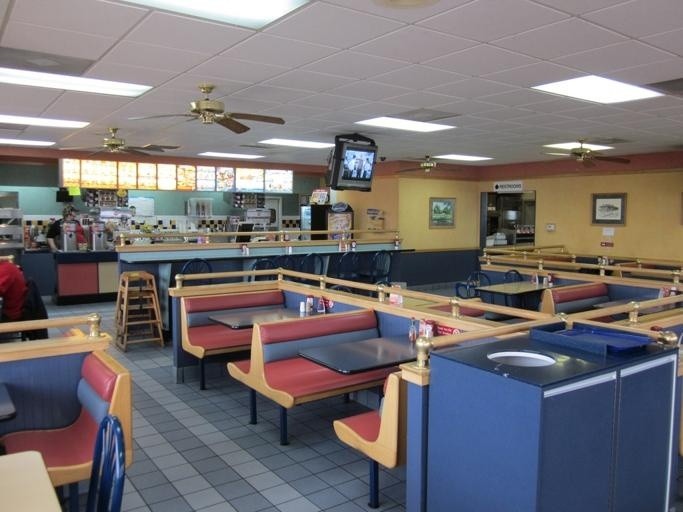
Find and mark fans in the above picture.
[127,82,286,136]
[538,138,630,162]
[395,155,462,175]
[58,127,180,157]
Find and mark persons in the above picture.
[341,154,372,181]
[46,205,87,254]
[130,206,137,218]
[0,259,28,322]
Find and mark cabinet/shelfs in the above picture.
[0,206,24,250]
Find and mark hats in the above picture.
[63,206,80,216]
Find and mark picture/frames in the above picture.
[429,197,457,228]
[592,193,627,224]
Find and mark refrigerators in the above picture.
[299,204,354,240]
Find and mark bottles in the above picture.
[280,231,289,241]
[670,286,677,297]
[543,272,553,287]
[409,317,434,342]
[242,244,249,255]
[394,235,399,249]
[299,294,326,313]
[390,285,402,304]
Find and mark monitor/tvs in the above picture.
[327,141,378,188]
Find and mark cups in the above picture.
[351,241,357,250]
